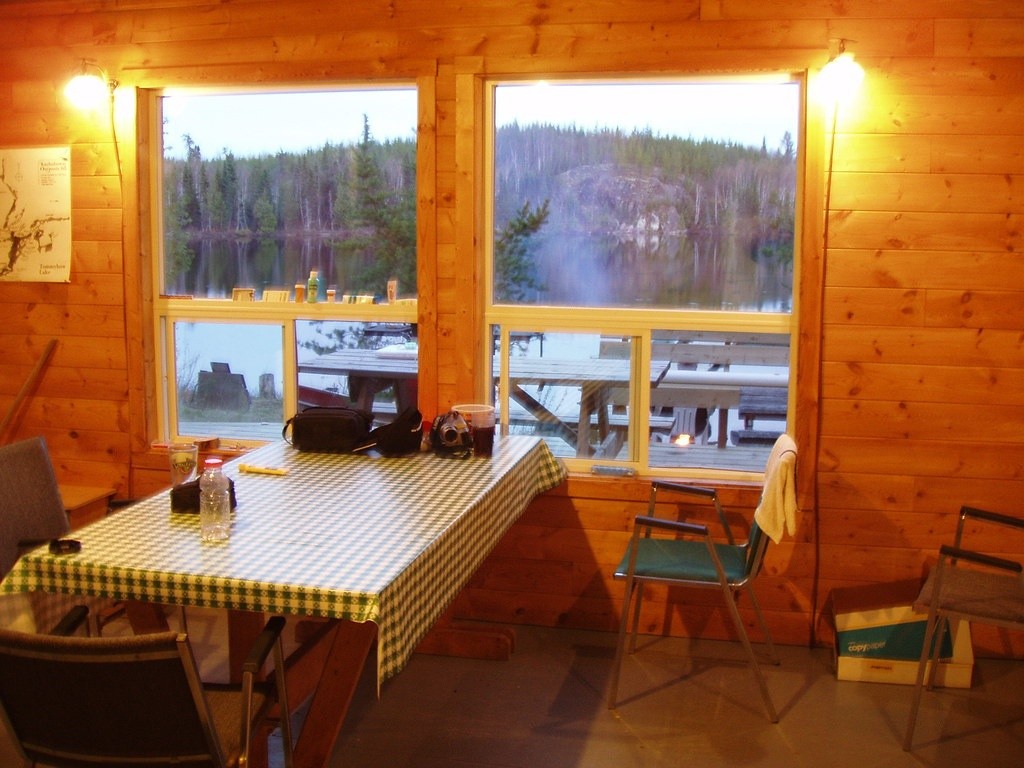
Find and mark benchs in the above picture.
[586,325,791,461]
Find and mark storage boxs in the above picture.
[832,576,955,660]
[833,616,974,690]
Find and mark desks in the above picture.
[297,348,673,460]
[0,434,570,768]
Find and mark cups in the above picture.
[168,445,198,491]
[451,404,495,447]
[294,285,306,303]
[472,412,495,458]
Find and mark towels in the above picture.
[753,433,799,544]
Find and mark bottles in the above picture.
[307,271,320,304]
[387,280,397,305]
[326,290,336,303]
[200,458,231,546]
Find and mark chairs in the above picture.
[0,435,295,768]
[902,506,1023,753]
[606,433,799,723]
